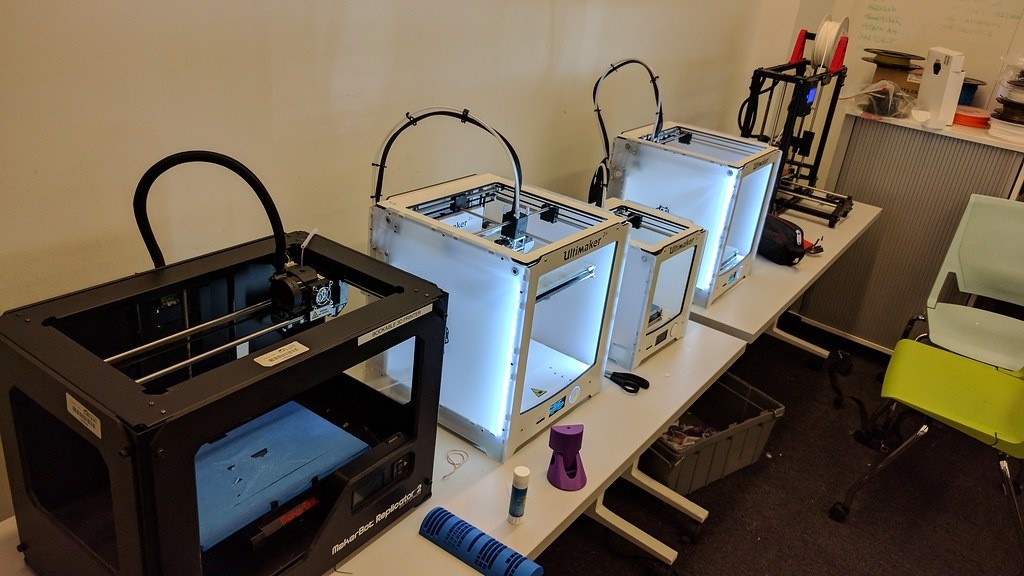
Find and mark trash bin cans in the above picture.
[645,370,786,496]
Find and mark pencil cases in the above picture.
[757,214,805,267]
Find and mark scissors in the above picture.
[603,371,649,394]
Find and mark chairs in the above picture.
[830,339,1024,543]
[880,194,1024,495]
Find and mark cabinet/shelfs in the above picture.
[788,115,1024,356]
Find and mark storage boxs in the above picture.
[637,371,785,495]
[915,47,966,125]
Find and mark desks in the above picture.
[0,320,748,576]
[689,185,883,359]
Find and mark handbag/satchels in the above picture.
[757,212,805,267]
